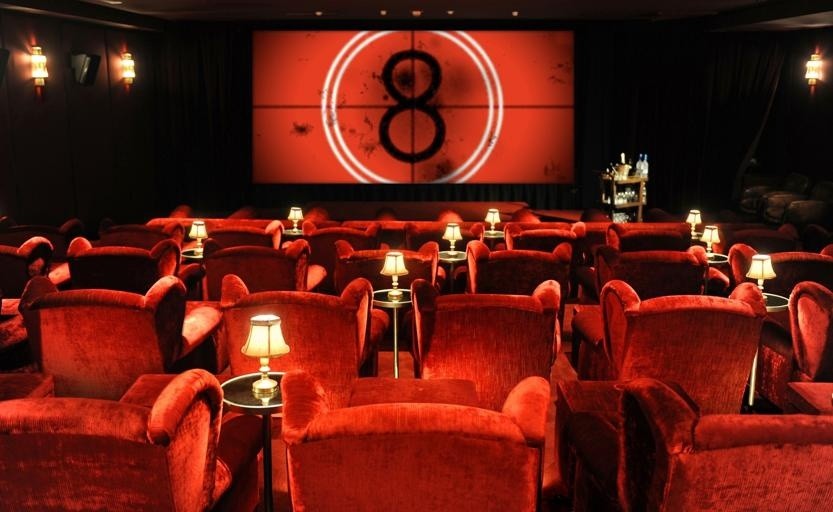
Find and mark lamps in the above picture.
[0,49,10,75]
[804,54,832,88]
[287,206,304,232]
[30,45,49,87]
[120,51,137,84]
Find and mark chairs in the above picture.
[1,210,833,511]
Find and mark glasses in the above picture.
[287,206,304,232]
[120,51,137,84]
[0,49,10,75]
[30,45,49,87]
[804,54,832,88]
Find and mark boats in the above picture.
[70,53,101,87]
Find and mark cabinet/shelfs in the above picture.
[607,152,651,180]
[602,173,649,222]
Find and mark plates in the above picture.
[602,173,649,222]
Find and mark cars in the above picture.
[608,185,646,225]
